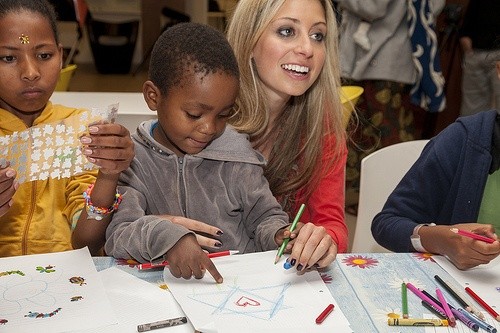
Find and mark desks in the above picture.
[49,89,160,137]
[2,251,500,331]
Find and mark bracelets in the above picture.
[84,183,124,221]
[411,222,437,254]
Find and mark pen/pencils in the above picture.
[401,274,500,333]
[134,250,242,272]
[450,228,495,243]
[274,204,307,265]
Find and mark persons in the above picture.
[1,0,136,259]
[371,110,500,271]
[154,1,360,276]
[103,22,307,284]
[336,0,447,215]
[458,0,500,120]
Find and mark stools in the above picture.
[85,16,141,76]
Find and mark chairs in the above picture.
[348,136,440,255]
